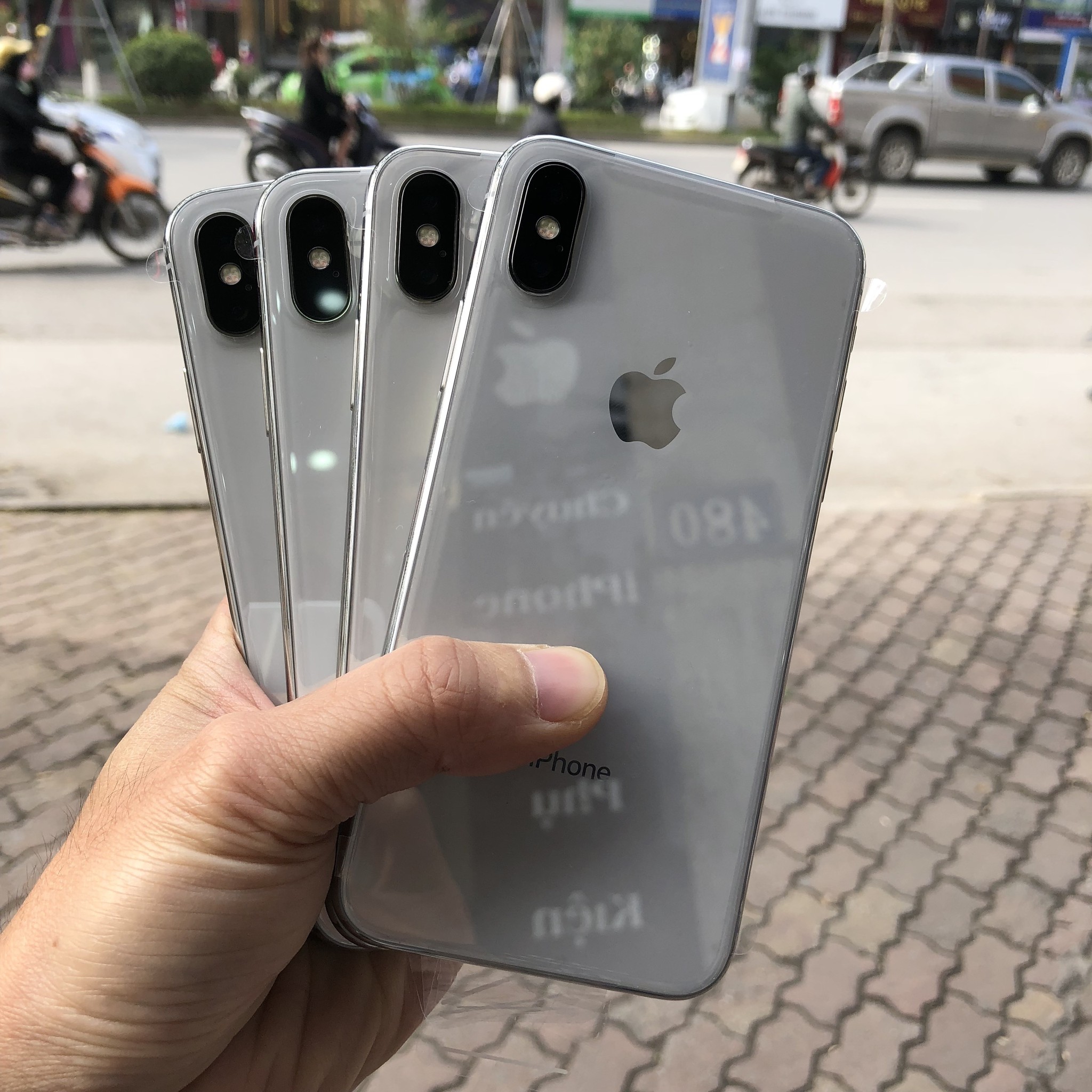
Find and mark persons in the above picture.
[0,37,87,241]
[0,591,608,1092]
[522,73,571,139]
[299,38,361,168]
[782,63,843,200]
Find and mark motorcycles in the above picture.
[0,118,174,264]
[239,91,400,184]
[735,121,874,219]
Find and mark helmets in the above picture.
[533,72,570,104]
[0,34,31,69]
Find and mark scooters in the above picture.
[206,56,283,106]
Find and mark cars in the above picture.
[33,91,162,193]
[276,42,460,117]
[776,49,1092,193]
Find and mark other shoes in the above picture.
[32,221,71,242]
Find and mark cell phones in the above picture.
[328,134,867,1001]
[161,134,502,955]
